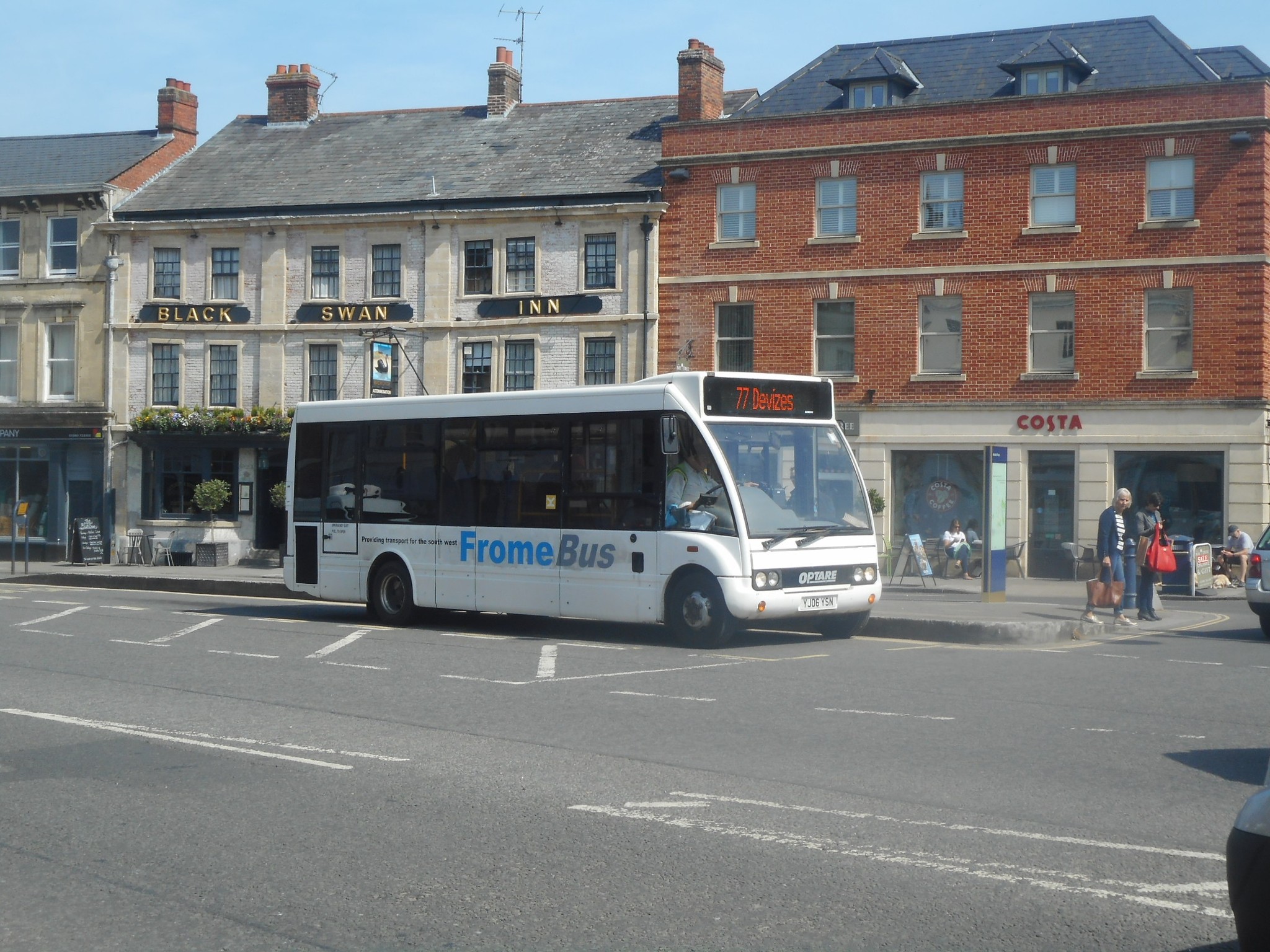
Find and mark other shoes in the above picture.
[955,563,960,569]
[1080,614,1103,624]
[1114,616,1137,627]
[1237,580,1245,587]
[963,575,973,580]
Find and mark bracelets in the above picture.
[1233,553,1235,558]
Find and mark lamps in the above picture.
[1225,128,1255,147]
[668,167,690,179]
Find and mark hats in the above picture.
[1227,525,1238,536]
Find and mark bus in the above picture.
[278,371,883,650]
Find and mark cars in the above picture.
[1244,522,1270,637]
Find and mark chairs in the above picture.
[882,534,897,578]
[1059,541,1096,580]
[1005,540,1027,579]
[150,530,177,568]
[878,553,890,579]
[943,556,971,580]
[909,536,943,579]
[123,528,146,565]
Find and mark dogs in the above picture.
[1195,573,1237,589]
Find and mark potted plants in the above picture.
[190,478,232,567]
[268,481,286,567]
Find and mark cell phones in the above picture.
[1160,520,1165,524]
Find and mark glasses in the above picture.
[953,526,960,528]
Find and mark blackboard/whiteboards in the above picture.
[69,517,105,563]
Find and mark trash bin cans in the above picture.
[1161,535,1194,595]
[165,551,194,567]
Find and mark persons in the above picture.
[784,467,796,511]
[1080,488,1137,627]
[1218,525,1254,587]
[942,518,973,579]
[964,519,979,549]
[1134,491,1167,622]
[664,443,760,518]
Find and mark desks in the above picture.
[125,534,155,566]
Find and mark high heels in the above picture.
[1137,611,1156,621]
[1149,609,1162,621]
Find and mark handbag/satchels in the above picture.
[1085,563,1125,608]
[1146,523,1176,573]
